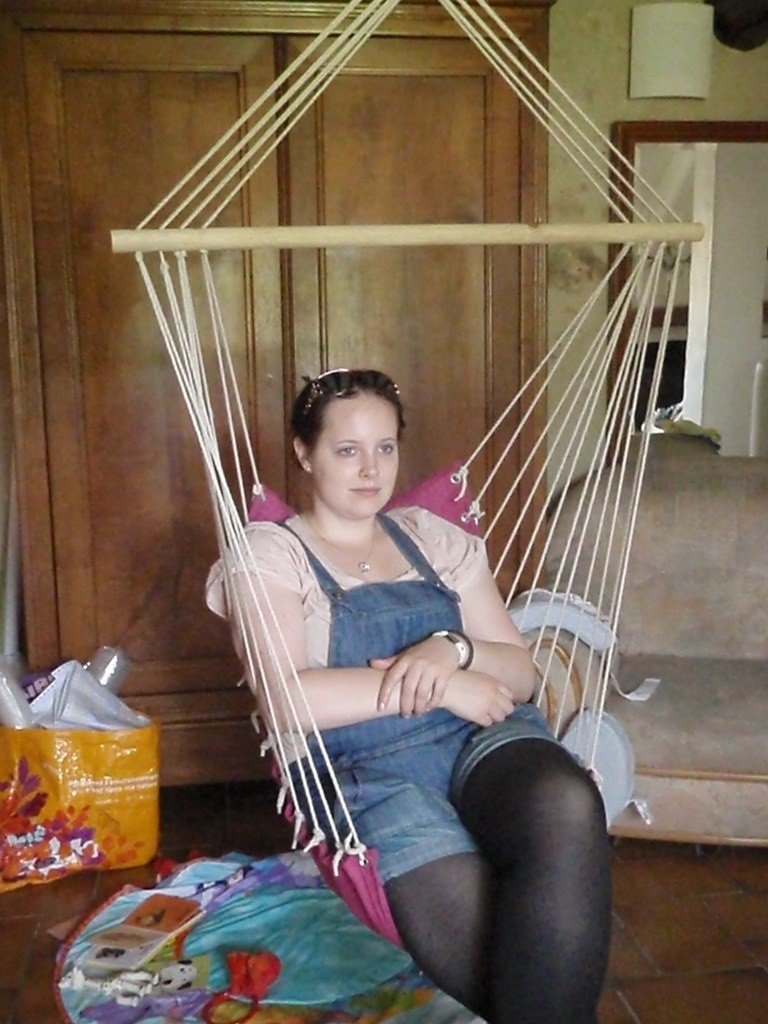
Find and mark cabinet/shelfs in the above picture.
[0,0,555,791]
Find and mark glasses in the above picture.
[296,369,403,433]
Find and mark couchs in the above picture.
[514,455,767,853]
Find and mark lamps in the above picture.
[628,2,716,99]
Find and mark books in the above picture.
[83,894,204,970]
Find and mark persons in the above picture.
[206,370,613,1024]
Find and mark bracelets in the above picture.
[433,630,473,669]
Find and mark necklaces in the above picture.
[307,517,376,572]
